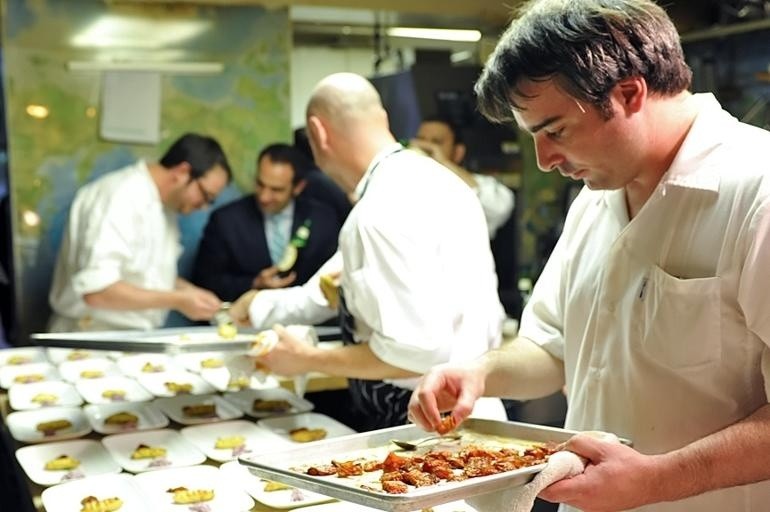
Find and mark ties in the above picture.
[270,214,284,264]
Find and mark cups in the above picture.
[214,302,242,341]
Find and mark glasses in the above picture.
[194,179,215,205]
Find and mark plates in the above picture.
[255,417,358,444]
[2,345,279,411]
[41,465,256,512]
[15,438,123,485]
[179,420,291,464]
[6,388,312,445]
[101,428,206,473]
[218,462,365,512]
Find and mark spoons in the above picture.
[390,433,466,453]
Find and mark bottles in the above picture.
[276,217,314,277]
[517,275,538,309]
[400,137,434,160]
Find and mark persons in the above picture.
[43,130,236,337]
[197,66,515,422]
[407,0,770,512]
